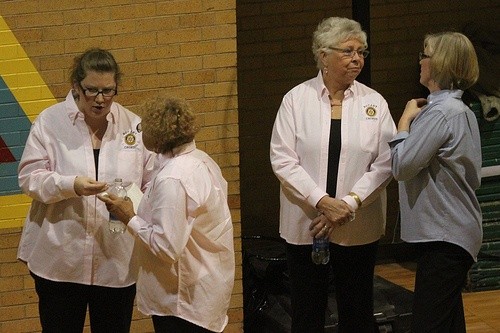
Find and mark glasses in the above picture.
[326,47,369,59]
[418,53,432,59]
[136,123,144,132]
[78,82,118,97]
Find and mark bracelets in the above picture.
[348,192,363,207]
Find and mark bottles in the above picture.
[108,178,127,234]
[311,234,330,264]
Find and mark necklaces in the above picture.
[331,104,342,113]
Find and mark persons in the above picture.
[388,31,483,333]
[16,46,157,333]
[96,92,236,333]
[270,17,398,333]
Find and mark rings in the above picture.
[324,226,327,229]
